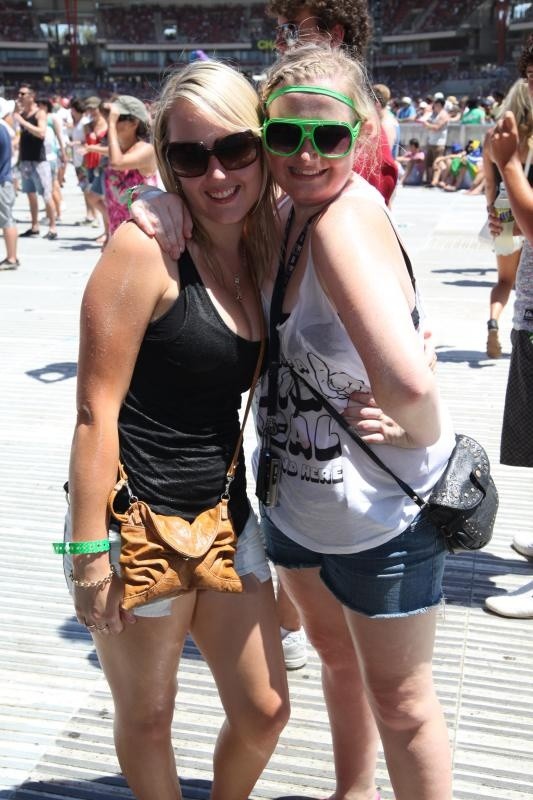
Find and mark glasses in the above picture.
[167,131,259,177]
[262,117,361,158]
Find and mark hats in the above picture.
[1,97,15,119]
[84,96,101,109]
[103,96,148,123]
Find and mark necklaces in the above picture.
[218,260,250,308]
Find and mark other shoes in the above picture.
[487,318,502,359]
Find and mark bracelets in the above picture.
[70,565,117,588]
[126,180,142,213]
[53,541,112,555]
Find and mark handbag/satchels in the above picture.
[120,501,243,610]
[428,435,498,551]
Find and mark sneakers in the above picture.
[485,581,533,618]
[76,218,92,226]
[90,218,100,229]
[281,626,307,669]
[512,534,533,557]
[41,231,58,242]
[20,229,39,237]
[0,259,20,271]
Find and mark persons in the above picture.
[482,53,533,622]
[252,0,398,671]
[123,42,454,800]
[0,85,167,271]
[352,84,500,196]
[62,60,290,800]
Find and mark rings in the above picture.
[86,625,96,633]
[98,627,107,632]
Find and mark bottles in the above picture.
[492,182,514,256]
